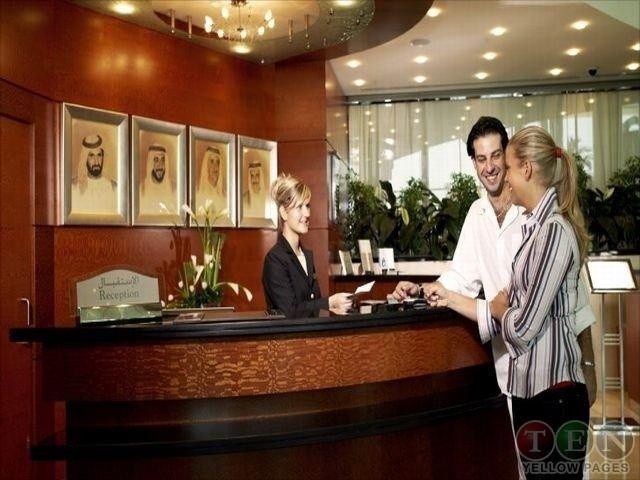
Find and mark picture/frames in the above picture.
[59,101,280,230]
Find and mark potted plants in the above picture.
[335,138,640,261]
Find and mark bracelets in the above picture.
[418,283,424,298]
[582,360,595,367]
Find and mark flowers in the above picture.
[159,201,253,309]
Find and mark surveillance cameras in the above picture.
[588,67,598,76]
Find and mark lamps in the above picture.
[204,1,276,40]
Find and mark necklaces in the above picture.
[495,197,510,218]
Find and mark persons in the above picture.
[260,173,355,315]
[244,160,268,218]
[195,145,228,216]
[387,115,598,408]
[423,126,588,479]
[139,143,177,215]
[72,134,118,213]
